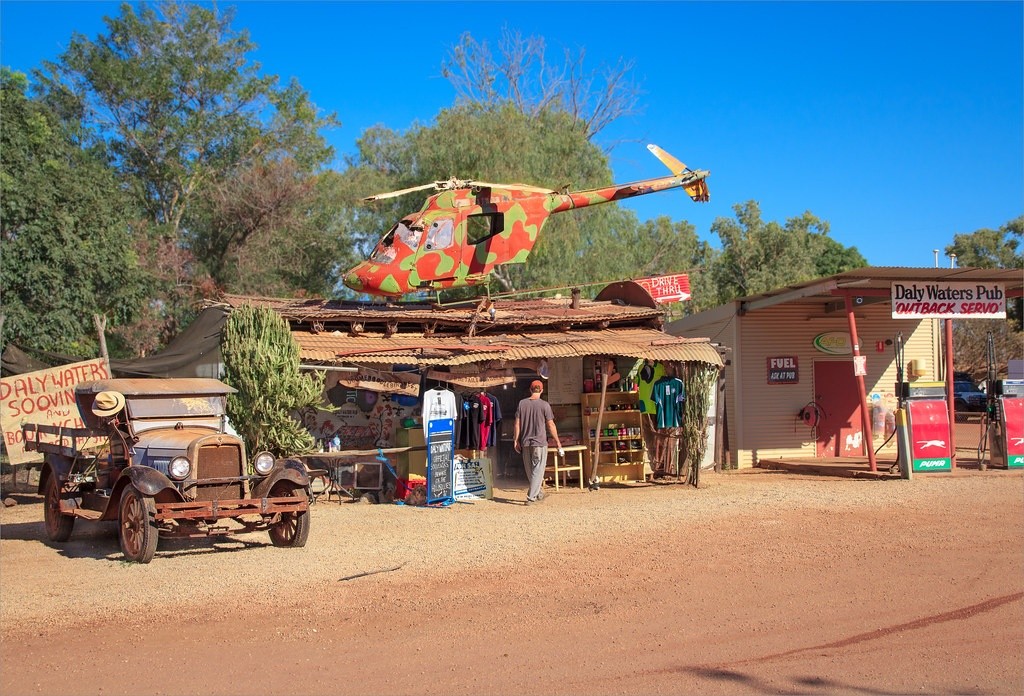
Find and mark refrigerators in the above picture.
[395,428,428,480]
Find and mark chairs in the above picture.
[300,457,328,505]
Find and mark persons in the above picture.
[513,380,565,505]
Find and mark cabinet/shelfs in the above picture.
[581,392,646,484]
[396,428,426,479]
[584,356,615,392]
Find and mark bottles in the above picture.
[316,435,340,453]
[596,369,600,373]
[618,378,638,391]
[596,359,601,366]
[596,374,601,381]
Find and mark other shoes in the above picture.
[538,493,549,501]
[525,499,533,505]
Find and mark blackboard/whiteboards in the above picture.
[428,435,454,502]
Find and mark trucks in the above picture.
[20,378,315,564]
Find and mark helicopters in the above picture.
[342,142,711,323]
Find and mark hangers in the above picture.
[434,381,487,397]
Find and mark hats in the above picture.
[531,380,543,390]
[92,391,125,416]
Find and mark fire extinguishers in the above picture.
[873,406,886,433]
[886,409,897,438]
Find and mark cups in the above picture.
[631,404,637,410]
[610,405,618,410]
[620,404,627,410]
[590,423,640,436]
[627,404,632,410]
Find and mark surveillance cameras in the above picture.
[856,297,863,303]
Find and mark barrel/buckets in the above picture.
[583,378,594,393]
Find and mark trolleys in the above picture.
[374,448,428,508]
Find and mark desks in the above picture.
[545,445,587,491]
[501,438,519,478]
[294,447,411,505]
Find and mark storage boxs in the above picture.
[396,479,427,500]
[355,462,382,490]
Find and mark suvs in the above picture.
[954,380,988,424]
